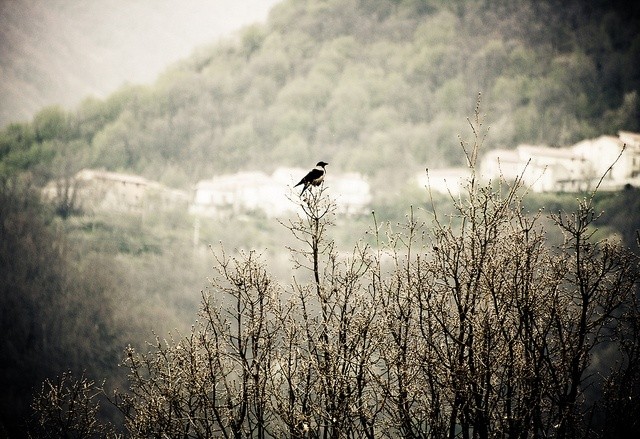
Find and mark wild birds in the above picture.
[294,161,330,197]
